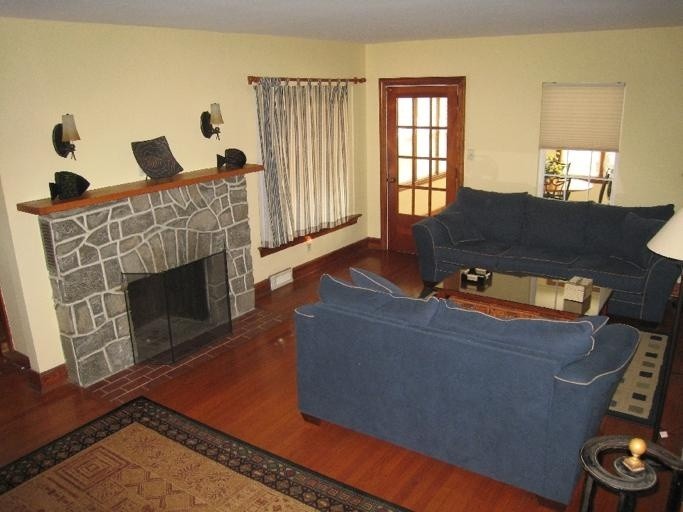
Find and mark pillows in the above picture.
[521,194,588,252]
[589,203,675,258]
[457,185,528,248]
[428,297,595,359]
[609,211,666,272]
[319,273,439,329]
[568,315,609,335]
[349,267,402,297]
[432,212,486,246]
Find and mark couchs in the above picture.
[411,186,683,324]
[293,274,642,505]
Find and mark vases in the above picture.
[544,176,560,192]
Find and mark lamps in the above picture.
[647,207,682,442]
[200,102,224,141]
[52,113,80,160]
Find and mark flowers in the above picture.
[543,148,564,176]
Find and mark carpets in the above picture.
[0,395,421,512]
[607,328,673,427]
